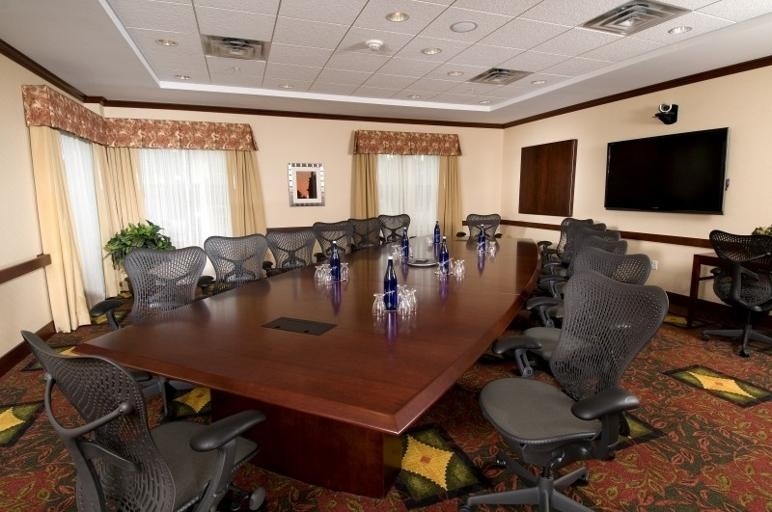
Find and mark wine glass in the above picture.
[476,241,496,259]
[391,244,405,261]
[435,257,465,278]
[313,262,349,283]
[373,284,420,318]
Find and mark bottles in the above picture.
[402,227,411,258]
[433,243,440,261]
[477,250,486,280]
[382,255,399,310]
[401,263,410,283]
[433,221,440,243]
[439,276,451,310]
[385,311,397,343]
[329,240,342,282]
[478,223,485,252]
[331,285,341,316]
[439,235,450,274]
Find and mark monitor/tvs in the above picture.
[604,126,730,216]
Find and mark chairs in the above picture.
[21,328,284,512]
[703,230,772,356]
[108,214,411,381]
[457,214,671,512]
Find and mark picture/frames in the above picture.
[288,162,325,206]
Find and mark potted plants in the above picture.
[102,219,175,298]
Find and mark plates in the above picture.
[406,258,436,267]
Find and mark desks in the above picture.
[686,255,771,327]
[72,236,541,500]
